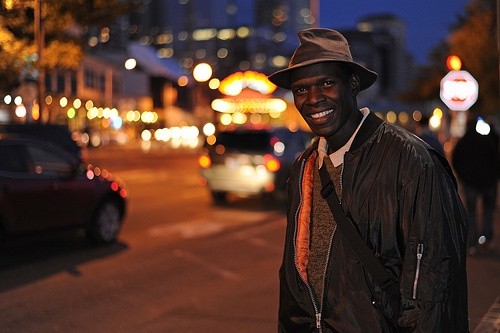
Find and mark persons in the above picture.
[413,116,444,153]
[269,28,469,333]
[452,110,500,256]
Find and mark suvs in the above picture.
[199,128,307,206]
[0,124,126,247]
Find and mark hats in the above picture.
[268,27,378,92]
